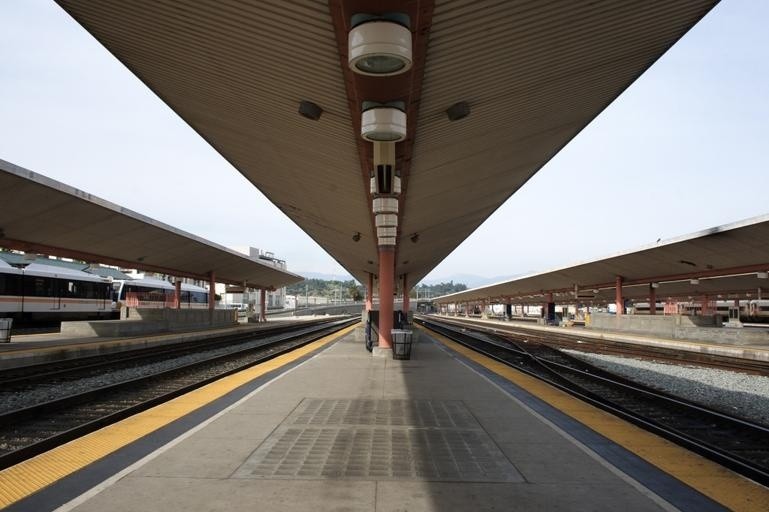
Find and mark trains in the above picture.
[2,257,220,332]
[626,298,769,327]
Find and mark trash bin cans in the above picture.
[390,329,414,360]
[0,318,14,343]
